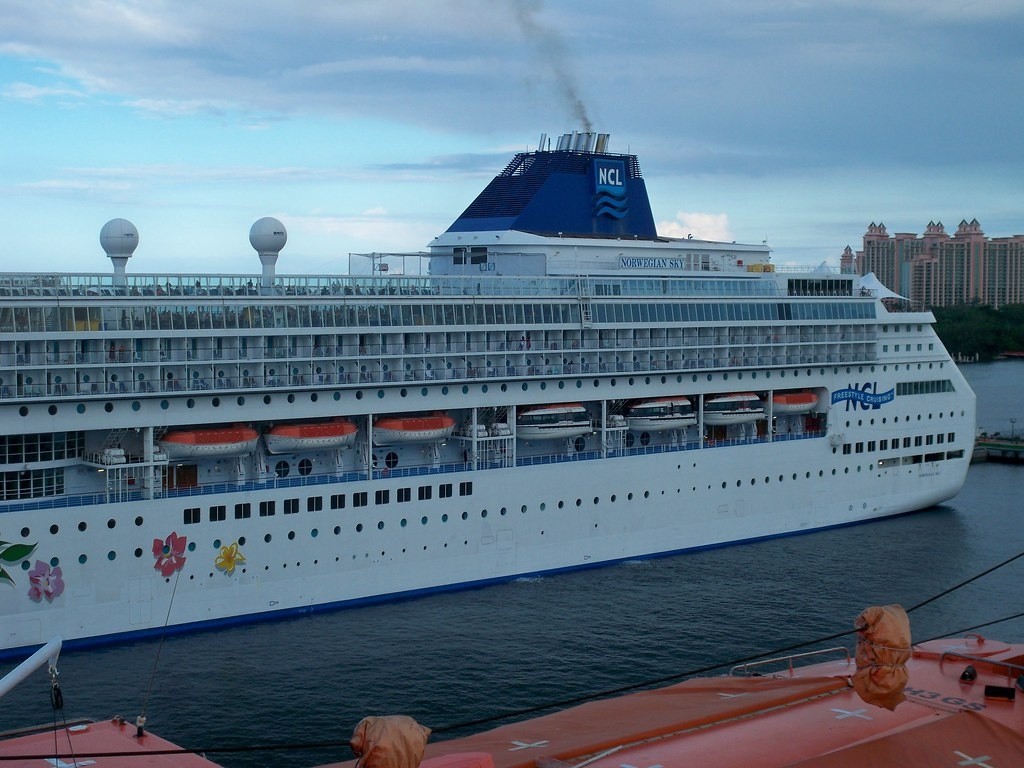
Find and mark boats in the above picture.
[515,402,594,440]
[622,396,698,429]
[371,414,457,444]
[2,129,978,655]
[771,392,820,415]
[703,393,764,423]
[149,421,260,459]
[261,420,360,454]
[970,417,1024,462]
[0,603,1023,768]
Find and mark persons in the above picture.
[518,336,525,351]
[526,335,531,350]
[508,336,514,350]
[76,279,492,330]
[107,345,116,362]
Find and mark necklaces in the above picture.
[117,345,127,363]
